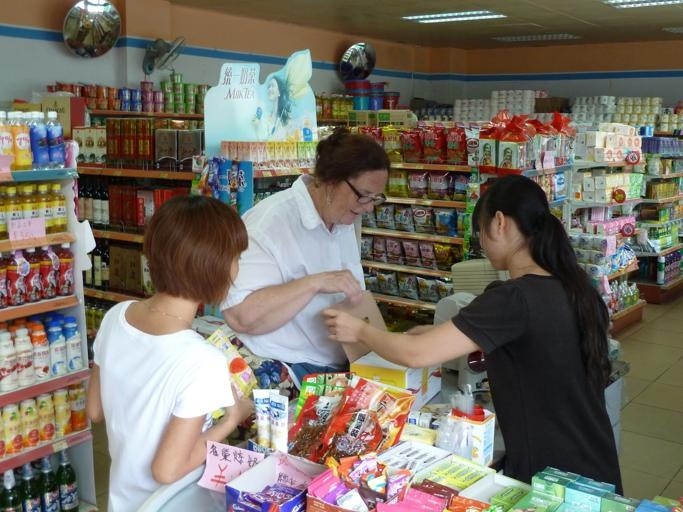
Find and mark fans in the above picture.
[142,35,187,75]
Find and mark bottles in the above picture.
[312,92,354,121]
[609,279,641,315]
[0,111,111,512]
[633,218,683,288]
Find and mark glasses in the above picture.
[343,178,386,206]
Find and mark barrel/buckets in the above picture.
[346,79,400,109]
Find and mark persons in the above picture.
[82,193,256,512]
[319,170,625,501]
[264,52,315,132]
[216,125,391,394]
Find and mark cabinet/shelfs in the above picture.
[57,108,572,335]
[566,112,681,337]
[0,109,95,512]
[136,335,631,512]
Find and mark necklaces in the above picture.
[139,299,190,326]
